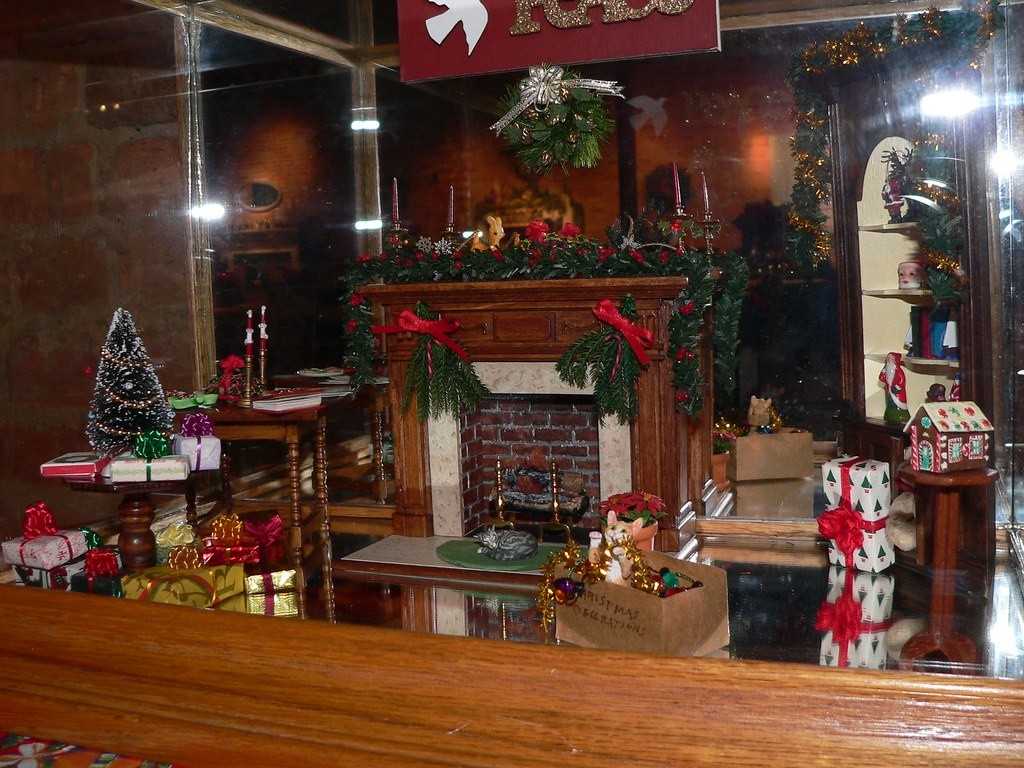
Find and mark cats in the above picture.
[588,510,645,589]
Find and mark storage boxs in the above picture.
[120,563,244,608]
[102,451,190,484]
[167,394,218,410]
[553,551,730,656]
[821,457,895,575]
[11,545,123,591]
[40,452,111,477]
[726,428,815,481]
[71,568,126,597]
[244,569,297,595]
[245,592,299,618]
[1,529,88,570]
[172,434,221,471]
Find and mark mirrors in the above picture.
[187,0,1014,562]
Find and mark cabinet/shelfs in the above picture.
[828,64,993,466]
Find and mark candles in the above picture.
[700,171,709,210]
[671,159,681,206]
[446,186,454,224]
[257,305,268,349]
[244,310,254,355]
[393,177,399,221]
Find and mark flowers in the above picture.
[594,488,668,529]
[712,428,737,455]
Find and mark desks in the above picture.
[60,472,202,570]
[172,400,336,621]
[273,374,391,504]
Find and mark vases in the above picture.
[711,452,730,493]
[599,520,658,552]
